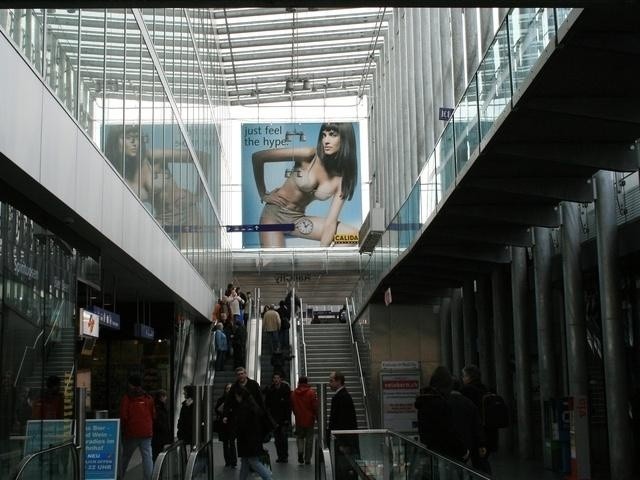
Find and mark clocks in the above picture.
[299,220,314,233]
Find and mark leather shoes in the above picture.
[282,458,288,463]
[276,457,282,462]
[298,455,303,463]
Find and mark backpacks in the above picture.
[479,391,509,428]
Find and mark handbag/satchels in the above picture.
[249,445,271,472]
[213,413,223,433]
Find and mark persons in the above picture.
[413,361,492,480]
[328,370,361,480]
[105,124,211,249]
[208,282,302,480]
[250,124,360,248]
[0,368,71,478]
[86,314,96,334]
[120,382,198,480]
[291,376,318,465]
